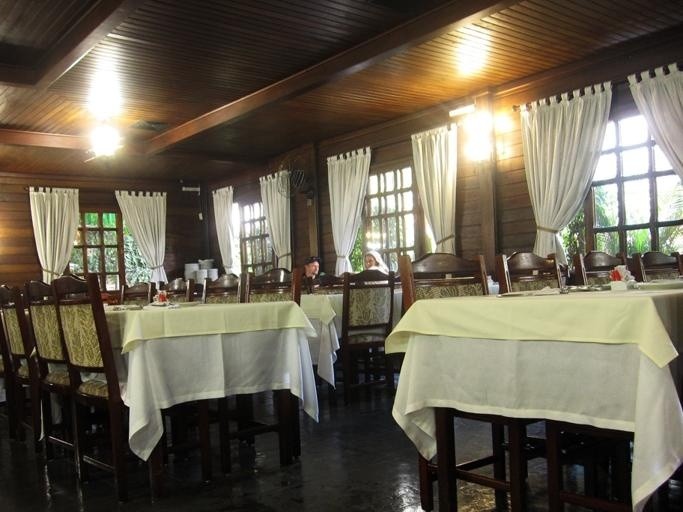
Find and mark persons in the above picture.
[300,256,321,289]
[364,250,389,273]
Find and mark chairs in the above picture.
[197,266,305,474]
[633,252,682,283]
[0,284,44,453]
[52,273,211,503]
[399,254,563,512]
[498,251,628,481]
[573,251,630,286]
[22,279,103,473]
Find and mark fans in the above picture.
[276,154,314,200]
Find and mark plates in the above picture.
[184,263,219,284]
